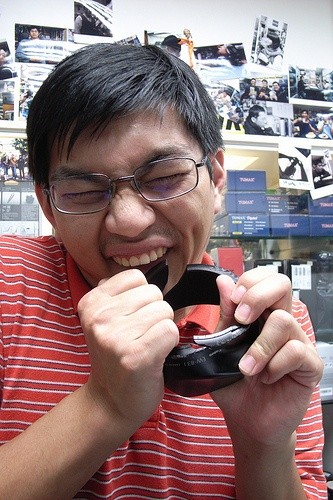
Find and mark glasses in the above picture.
[42,155,208,215]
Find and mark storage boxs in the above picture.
[214,169,333,235]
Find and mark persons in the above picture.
[0,40,329,500]
[163,26,333,190]
[75,5,99,37]
[20,26,46,62]
[0,41,18,80]
[4,156,24,181]
